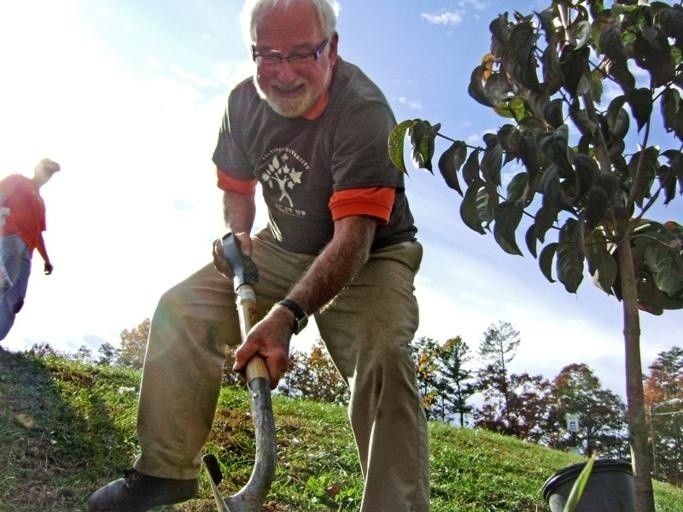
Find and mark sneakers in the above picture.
[86,467,200,512]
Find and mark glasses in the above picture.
[250,37,331,70]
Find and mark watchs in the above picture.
[278,298,308,335]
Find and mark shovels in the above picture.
[200,232,276,512]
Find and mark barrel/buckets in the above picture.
[541,457,638,511]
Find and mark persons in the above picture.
[0,159,61,341]
[87,1,431,511]
